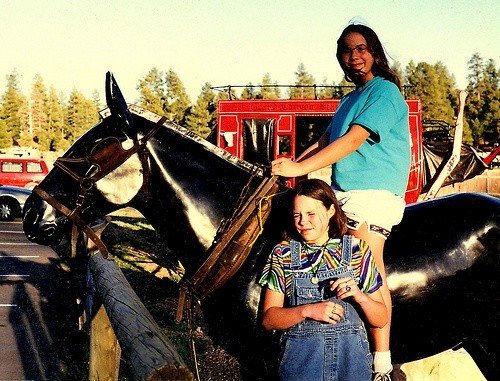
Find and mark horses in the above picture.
[23,71,500,381]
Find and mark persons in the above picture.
[271,24,411,381]
[259,178,388,381]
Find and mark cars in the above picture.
[0,184,33,221]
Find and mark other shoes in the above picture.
[373,365,393,381]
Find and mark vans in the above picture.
[0,157,49,191]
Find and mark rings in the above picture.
[330,313,334,318]
[334,302,336,306]
[345,282,348,287]
[346,287,351,292]
[327,318,331,323]
[332,307,336,313]
